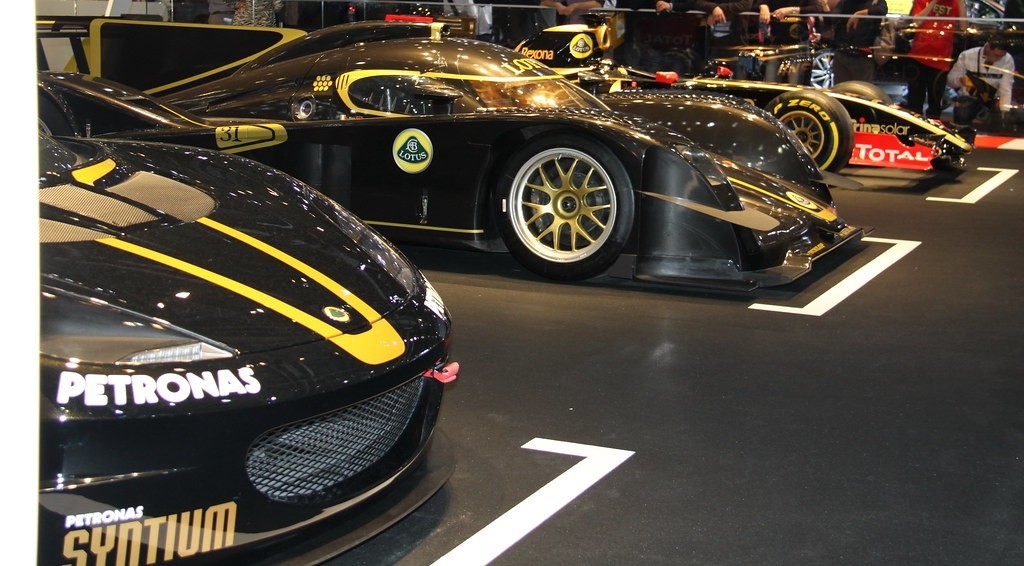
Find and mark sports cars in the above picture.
[40,115,459,565]
[38,15,978,194]
[39,31,869,302]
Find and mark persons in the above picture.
[223,0,284,28]
[755,0,1024,131]
[469,0,757,79]
